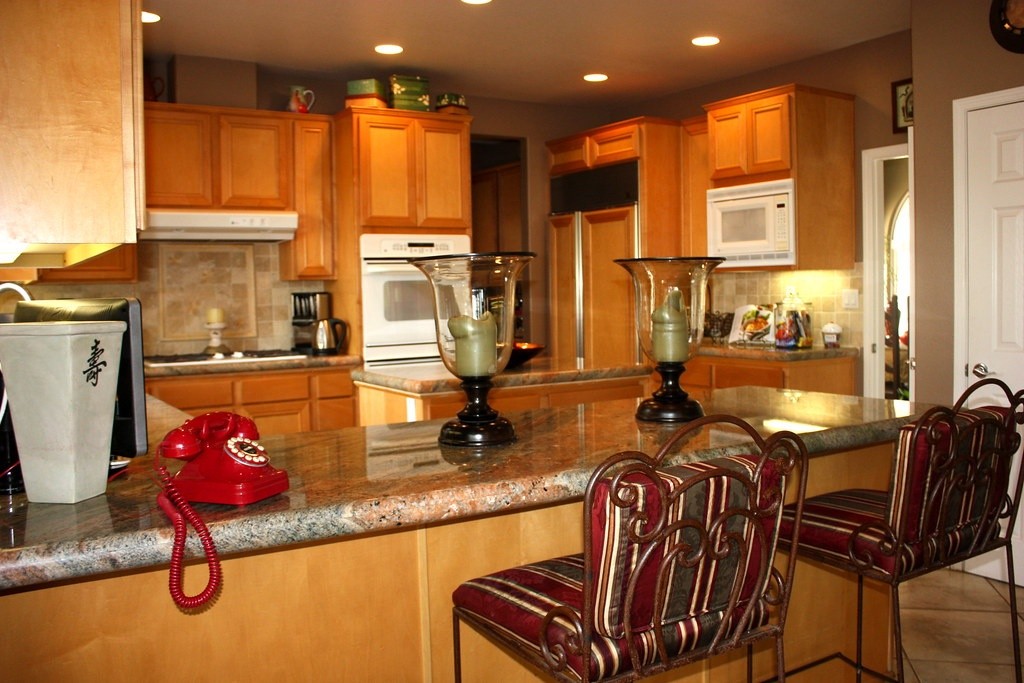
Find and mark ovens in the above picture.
[360,233,472,362]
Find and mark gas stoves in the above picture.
[142,349,308,367]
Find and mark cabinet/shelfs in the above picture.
[354,376,649,426]
[472,161,523,254]
[143,101,288,210]
[334,109,474,228]
[145,365,360,437]
[651,355,859,395]
[280,112,335,282]
[0,0,146,270]
[701,83,856,180]
[544,116,682,363]
[41,244,138,283]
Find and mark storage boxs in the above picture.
[435,93,469,115]
[345,79,388,108]
[389,75,430,113]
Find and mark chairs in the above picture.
[747,378,1024,683]
[451,415,809,681]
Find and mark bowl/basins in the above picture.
[497,342,547,370]
[899,337,908,346]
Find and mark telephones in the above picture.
[161,410,290,508]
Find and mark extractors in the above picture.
[137,209,299,244]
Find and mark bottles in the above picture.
[821,322,843,349]
[774,292,813,349]
[286,83,315,113]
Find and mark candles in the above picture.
[206,308,224,323]
[651,290,689,362]
[448,311,497,376]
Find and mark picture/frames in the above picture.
[891,78,914,134]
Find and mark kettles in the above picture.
[310,318,346,356]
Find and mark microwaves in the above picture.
[706,177,796,268]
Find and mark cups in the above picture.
[1,320,128,504]
